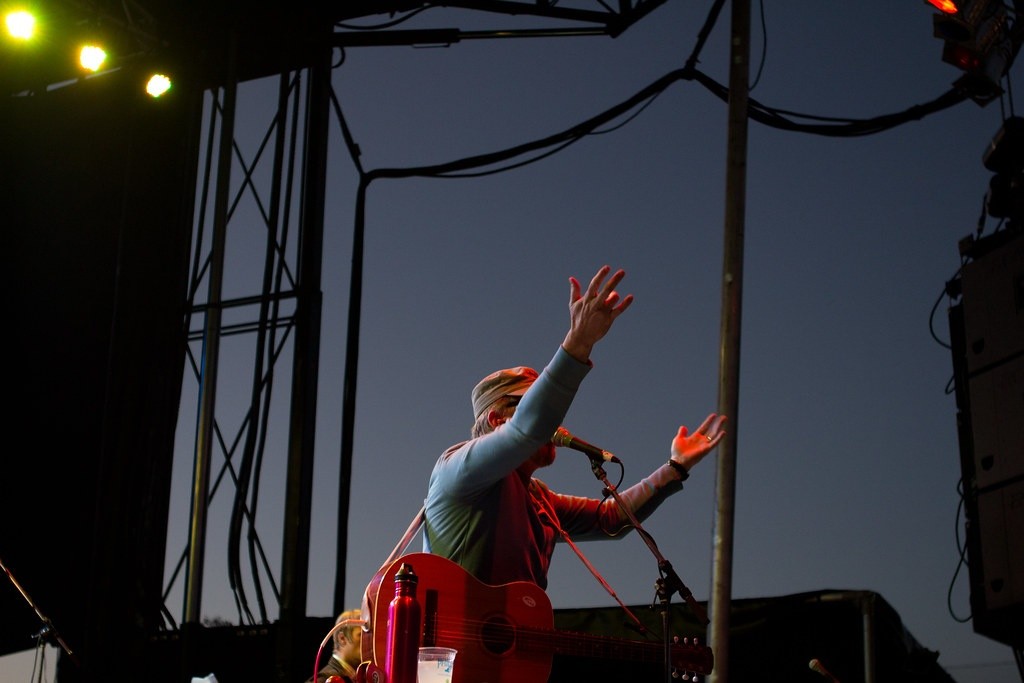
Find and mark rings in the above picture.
[706,435,713,441]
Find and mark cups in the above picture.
[417,646,458,683]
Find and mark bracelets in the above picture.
[668,458,689,482]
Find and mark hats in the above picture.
[471,367,538,420]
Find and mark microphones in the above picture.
[550,427,620,463]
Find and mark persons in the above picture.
[304,609,363,683]
[418,263,728,591]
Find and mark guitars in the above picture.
[361,551,711,682]
